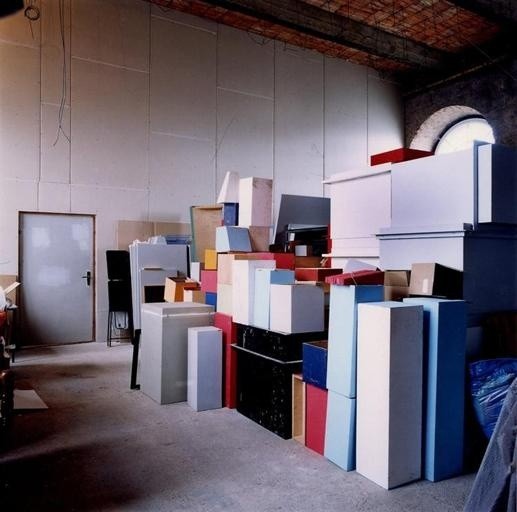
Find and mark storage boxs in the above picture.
[164,275,189,303]
[215,225,251,253]
[230,323,327,441]
[190,262,204,282]
[204,248,218,270]
[302,340,329,390]
[274,252,295,269]
[326,285,386,397]
[324,270,384,286]
[248,225,274,252]
[183,289,205,302]
[402,296,466,482]
[254,269,294,330]
[305,383,327,457]
[322,390,357,473]
[292,373,306,446]
[138,302,216,406]
[295,267,343,281]
[205,292,217,312]
[270,284,325,334]
[356,300,424,491]
[408,262,464,299]
[216,284,233,314]
[216,171,239,224]
[238,176,273,226]
[218,253,274,285]
[384,269,411,301]
[200,270,216,293]
[232,259,277,325]
[187,326,223,412]
